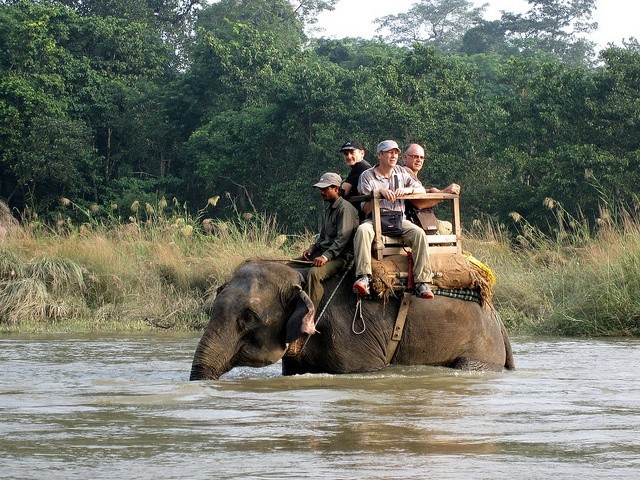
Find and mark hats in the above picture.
[377,140,402,153]
[314,173,341,188]
[342,140,363,150]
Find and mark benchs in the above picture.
[340,188,461,260]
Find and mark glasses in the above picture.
[407,153,425,159]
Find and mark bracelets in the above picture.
[322,255,329,262]
[402,143,461,246]
[412,189,414,195]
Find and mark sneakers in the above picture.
[416,283,434,299]
[352,276,371,296]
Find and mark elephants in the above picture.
[189,256,515,381]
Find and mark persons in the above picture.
[351,139,434,299]
[339,140,373,222]
[286,172,360,335]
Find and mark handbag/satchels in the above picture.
[380,208,403,237]
[415,209,439,235]
